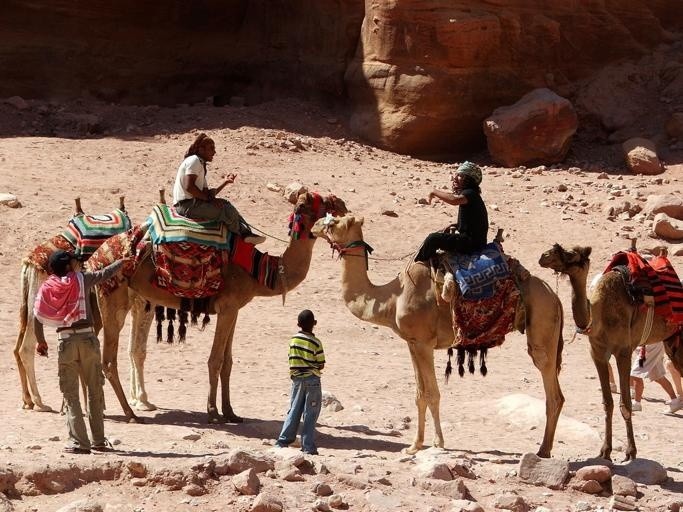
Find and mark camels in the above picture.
[537,242,683,462]
[307,214,567,458]
[84,182,353,428]
[12,206,135,415]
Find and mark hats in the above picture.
[457,161,482,184]
[50,250,72,266]
[195,133,214,147]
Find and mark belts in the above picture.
[57,327,95,340]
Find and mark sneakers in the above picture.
[630,386,642,411]
[242,232,266,243]
[598,383,617,393]
[65,443,104,454]
[664,396,683,414]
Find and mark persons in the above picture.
[596,354,636,398]
[172,133,268,246]
[629,339,676,417]
[662,354,682,415]
[33,246,137,454]
[273,309,327,457]
[412,160,489,265]
